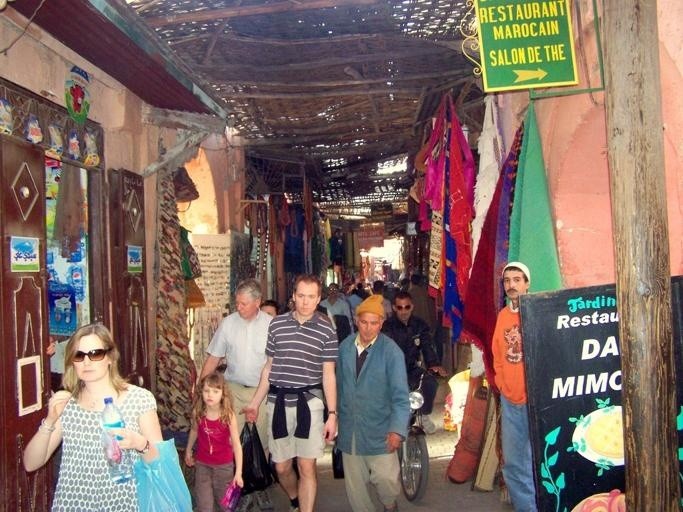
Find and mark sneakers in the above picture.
[233,493,254,512]
[255,488,274,509]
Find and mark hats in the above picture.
[501,262,530,283]
[356,295,385,318]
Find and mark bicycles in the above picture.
[398,370,443,505]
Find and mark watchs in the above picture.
[139,441,150,454]
[328,411,338,416]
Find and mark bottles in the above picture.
[100,396,133,485]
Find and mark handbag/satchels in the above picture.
[179,226,201,280]
[48,280,76,336]
[173,167,199,202]
[184,279,206,307]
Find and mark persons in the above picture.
[241,274,337,512]
[183,373,244,512]
[23,324,163,512]
[491,261,537,512]
[199,278,274,512]
[336,294,410,511]
[321,278,447,435]
[261,300,281,317]
[46,337,56,357]
[286,298,295,309]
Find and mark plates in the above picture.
[570,404,626,467]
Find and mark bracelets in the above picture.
[39,418,56,433]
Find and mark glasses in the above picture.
[73,348,112,362]
[395,304,414,311]
